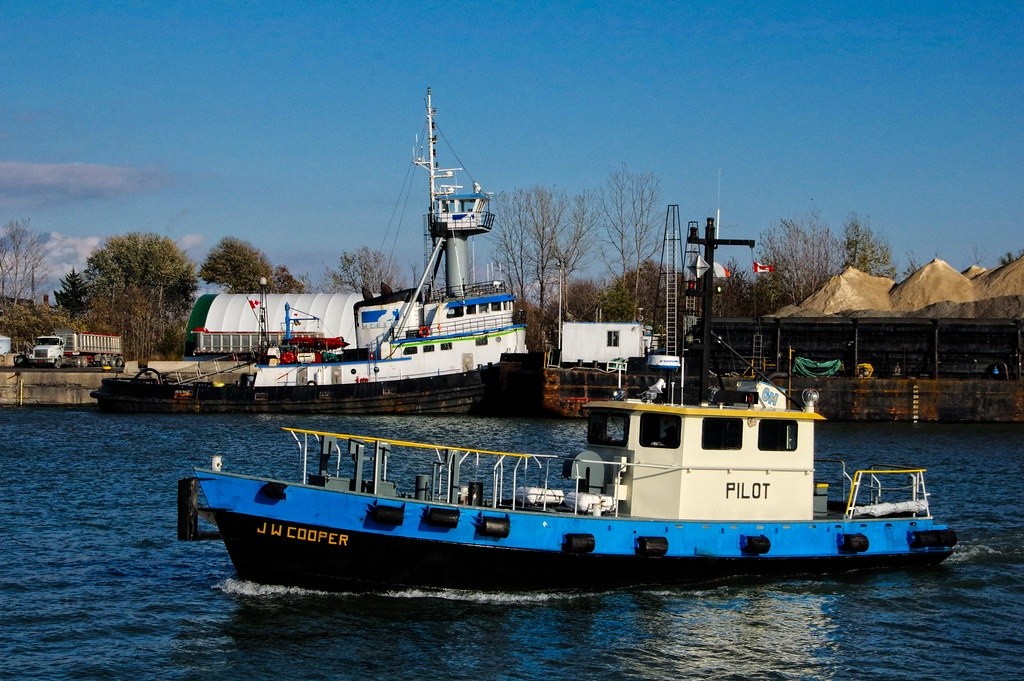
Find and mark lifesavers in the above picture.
[419,325,431,338]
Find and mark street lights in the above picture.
[556,262,562,366]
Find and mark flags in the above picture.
[249,300,261,310]
[370,352,374,360]
[753,261,775,273]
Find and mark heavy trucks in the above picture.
[27,330,124,369]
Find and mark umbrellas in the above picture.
[191,326,207,351]
[713,261,730,277]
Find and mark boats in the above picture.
[514,484,563,506]
[167,390,201,414]
[193,218,957,593]
[90,84,547,414]
[499,218,1024,421]
[562,492,618,515]
[290,336,351,350]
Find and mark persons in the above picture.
[267,340,280,367]
[193,346,199,356]
